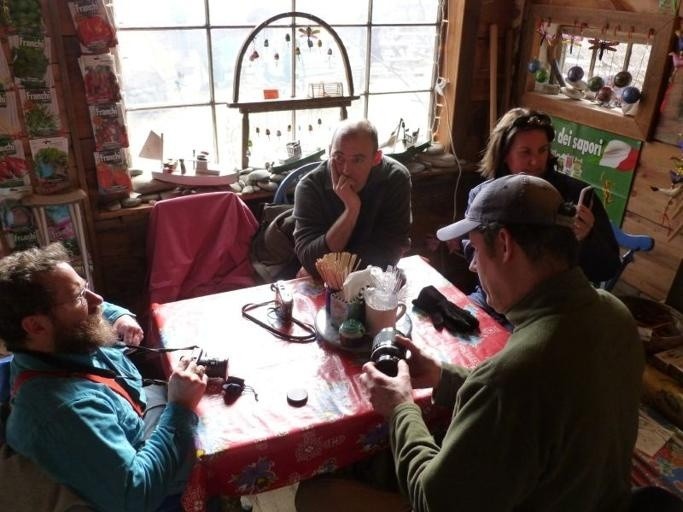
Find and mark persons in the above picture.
[292,170,644,511]
[292,115,414,285]
[461,107,621,335]
[1,242,211,512]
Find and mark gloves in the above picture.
[412,285,482,341]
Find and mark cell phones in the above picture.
[580,186,594,210]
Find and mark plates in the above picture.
[314,305,411,353]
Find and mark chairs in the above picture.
[597,223,653,296]
[272,158,327,210]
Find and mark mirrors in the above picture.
[522,5,674,143]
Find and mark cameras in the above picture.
[188,346,230,385]
[275,280,293,320]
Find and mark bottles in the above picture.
[372,326,405,374]
[340,319,367,347]
[162,151,219,176]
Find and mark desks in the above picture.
[132,247,512,511]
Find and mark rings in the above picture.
[195,365,204,376]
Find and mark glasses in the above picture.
[43,281,92,309]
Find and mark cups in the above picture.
[263,89,279,100]
[306,82,343,98]
[323,281,340,314]
[365,302,405,338]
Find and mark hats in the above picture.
[434,172,577,242]
